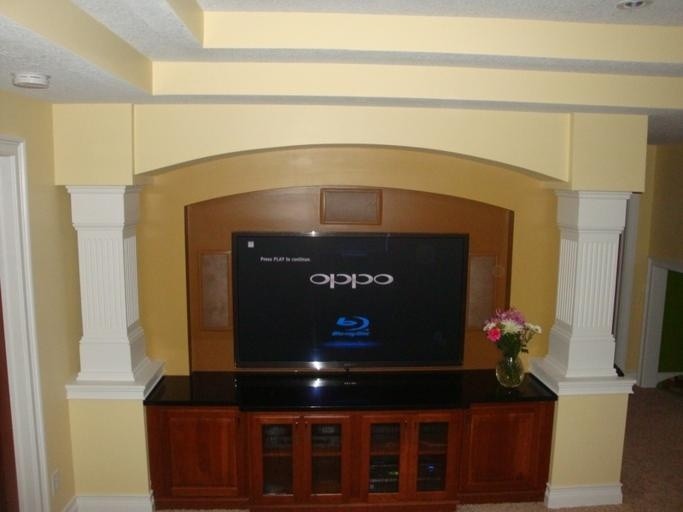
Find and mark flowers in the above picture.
[481,308,543,352]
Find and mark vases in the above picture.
[494,342,525,388]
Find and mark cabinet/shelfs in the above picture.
[141,366,565,510]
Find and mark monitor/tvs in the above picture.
[230,231,470,368]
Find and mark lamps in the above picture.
[615,0,656,13]
[8,71,52,92]
